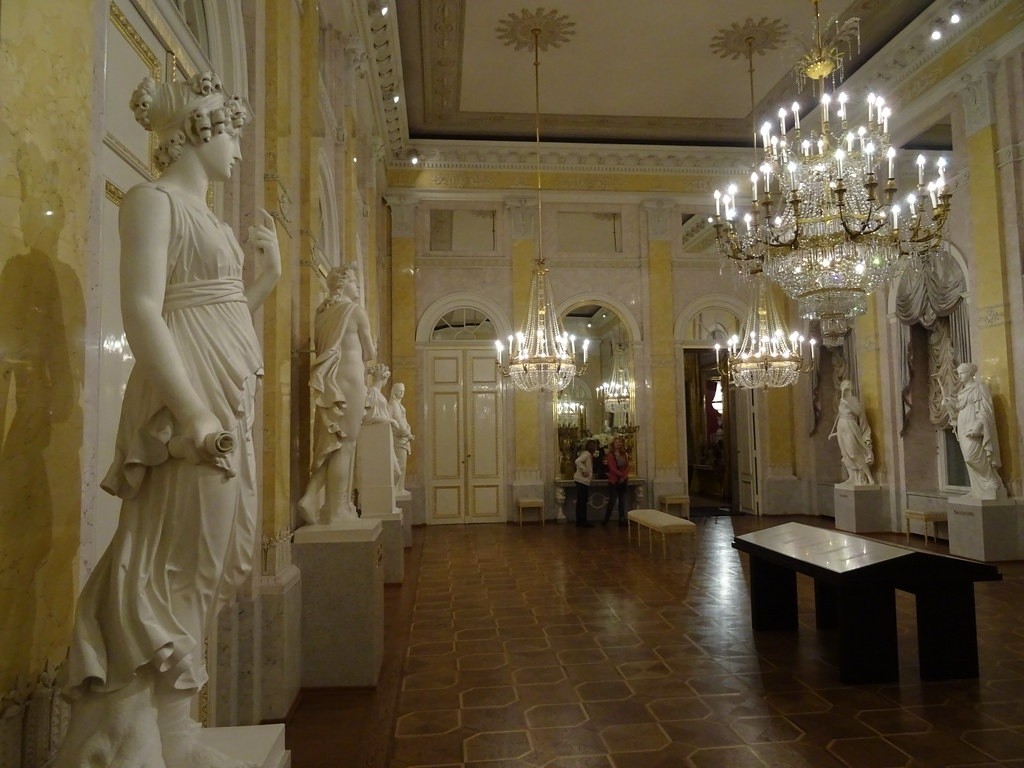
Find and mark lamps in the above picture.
[595,342,634,413]
[410,152,418,165]
[494,6,590,391]
[393,96,400,103]
[378,6,388,16]
[712,0,952,348]
[602,313,607,318]
[711,18,816,388]
[929,24,944,41]
[949,9,962,24]
[558,388,584,428]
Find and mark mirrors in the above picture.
[559,305,634,478]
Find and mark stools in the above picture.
[661,495,689,521]
[517,498,544,528]
[904,508,947,550]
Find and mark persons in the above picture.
[941,363,1007,500]
[37,71,281,768]
[828,380,875,485]
[573,440,599,528]
[297,264,375,524]
[360,363,401,487]
[712,440,726,487]
[601,438,628,528]
[388,382,416,494]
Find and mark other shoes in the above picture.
[602,520,608,526]
[576,521,595,528]
[619,521,628,526]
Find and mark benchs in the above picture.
[627,509,699,561]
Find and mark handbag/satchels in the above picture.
[618,477,628,484]
